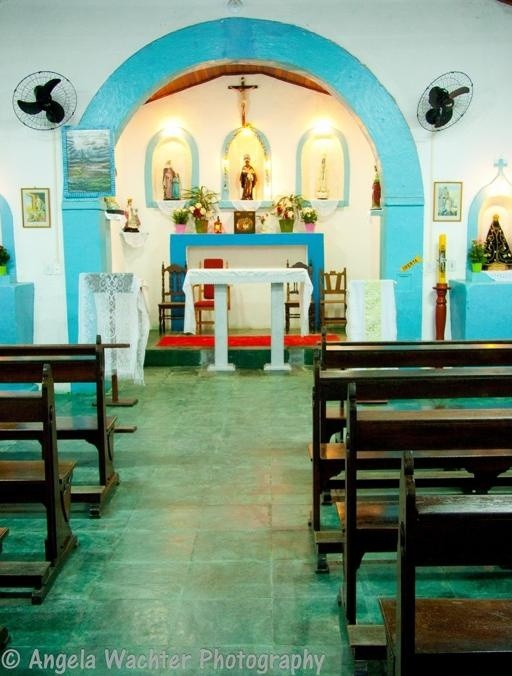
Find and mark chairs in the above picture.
[156,256,399,342]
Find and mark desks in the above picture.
[167,232,325,331]
[183,266,314,376]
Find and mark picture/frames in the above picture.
[20,186,50,228]
[433,181,461,222]
[61,124,115,199]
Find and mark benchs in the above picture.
[0,336,138,605]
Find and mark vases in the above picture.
[174,220,208,234]
[278,218,314,232]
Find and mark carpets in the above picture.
[155,335,341,348]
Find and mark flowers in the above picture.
[170,186,216,224]
[270,194,317,224]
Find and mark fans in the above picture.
[416,69,473,133]
[10,68,75,131]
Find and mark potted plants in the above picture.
[0,245,11,276]
[464,239,488,272]
